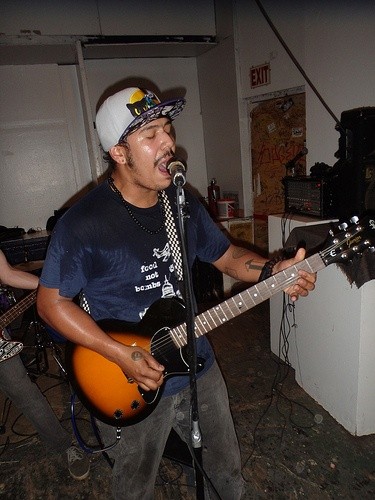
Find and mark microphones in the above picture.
[285,148,309,166]
[167,160,186,188]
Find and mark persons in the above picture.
[36,86,317,500]
[0,249,90,479]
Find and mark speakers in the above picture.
[192,260,224,301]
[338,106,375,221]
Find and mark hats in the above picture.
[94,85,186,152]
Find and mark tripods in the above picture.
[14,249,68,380]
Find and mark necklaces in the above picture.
[107,172,166,235]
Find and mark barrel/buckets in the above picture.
[217,199,236,219]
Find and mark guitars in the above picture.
[63,215,375,429]
[0,288,44,363]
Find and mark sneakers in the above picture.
[61,446,92,479]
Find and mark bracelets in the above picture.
[258,255,286,282]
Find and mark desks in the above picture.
[264,214,375,437]
